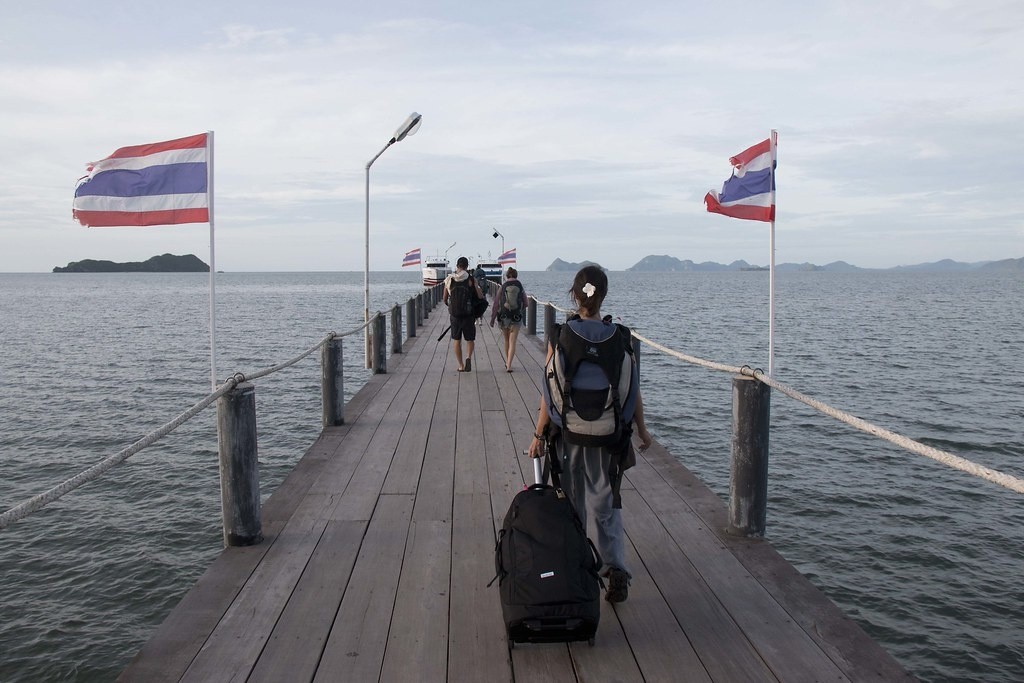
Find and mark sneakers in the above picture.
[606,569,629,603]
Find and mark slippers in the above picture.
[466,357,471,371]
[456,367,465,373]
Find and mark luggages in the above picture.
[488,445,604,648]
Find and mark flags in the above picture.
[703,131,780,222]
[72,131,210,227]
[401,248,421,266]
[498,247,517,264]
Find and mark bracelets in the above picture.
[534,432,546,440]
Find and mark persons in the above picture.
[442,256,489,372]
[528,265,651,603]
[489,267,528,373]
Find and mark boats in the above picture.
[477,259,502,285]
[421,255,452,287]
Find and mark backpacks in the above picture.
[544,318,642,446]
[473,269,488,297]
[449,273,476,317]
[500,279,524,322]
